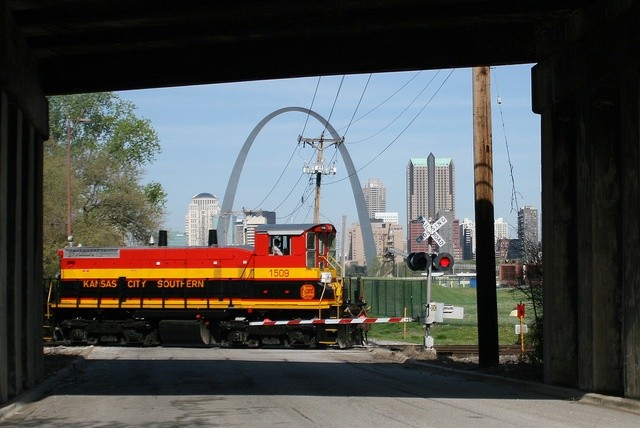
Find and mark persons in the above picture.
[271,239,283,255]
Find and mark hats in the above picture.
[275,238,281,242]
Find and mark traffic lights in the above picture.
[406,250,431,271]
[429,253,454,272]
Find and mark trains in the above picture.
[43,223,372,348]
